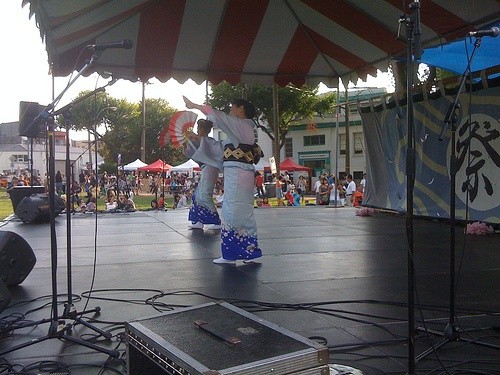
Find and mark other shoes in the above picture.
[188,221,202,228]
[243,258,263,263]
[208,225,219,229]
[213,257,236,264]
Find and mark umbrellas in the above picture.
[119,158,200,172]
[264,158,310,183]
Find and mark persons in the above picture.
[0,169,225,214]
[183,95,263,264]
[254,170,366,207]
[181,119,222,230]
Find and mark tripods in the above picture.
[158,160,175,211]
[412,37,500,366]
[0,79,116,340]
[0,47,121,357]
[60,142,95,215]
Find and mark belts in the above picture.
[346,194,352,196]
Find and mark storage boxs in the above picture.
[127,300,330,375]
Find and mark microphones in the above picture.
[111,75,138,82]
[88,40,133,50]
[161,156,164,160]
[468,26,500,37]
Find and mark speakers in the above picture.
[0,231,36,314]
[15,192,65,223]
[18,101,40,137]
